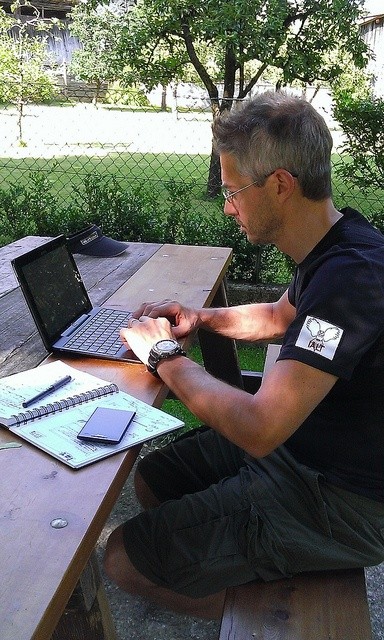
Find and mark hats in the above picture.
[64,224,130,259]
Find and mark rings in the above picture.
[129,319,138,326]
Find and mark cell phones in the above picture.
[76,406,136,446]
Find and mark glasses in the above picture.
[220,169,298,204]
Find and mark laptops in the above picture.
[11,235,143,363]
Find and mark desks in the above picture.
[0,236,233,639]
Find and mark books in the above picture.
[0,360,185,470]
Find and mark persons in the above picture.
[105,92,384,618]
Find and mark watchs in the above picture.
[146,339,186,379]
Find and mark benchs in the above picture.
[218,341,376,640]
